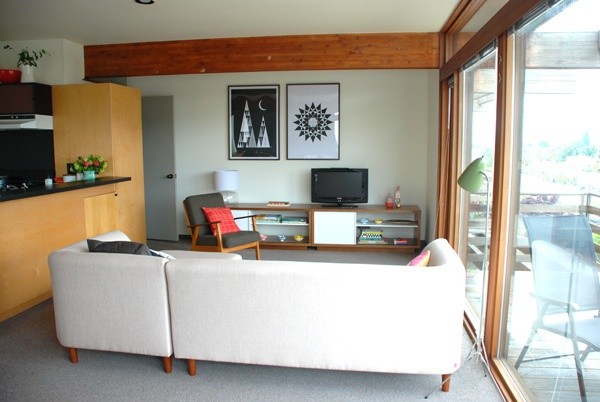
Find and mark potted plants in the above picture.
[3,43,50,82]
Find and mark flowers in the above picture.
[73,153,108,172]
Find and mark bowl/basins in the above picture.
[278,235,286,242]
[360,218,369,225]
[260,235,268,240]
[294,235,304,242]
[0,69,21,83]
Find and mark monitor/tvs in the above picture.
[310,167,369,208]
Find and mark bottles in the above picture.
[386,193,393,208]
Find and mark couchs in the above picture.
[165,237,467,392]
[46,229,243,374]
[182,191,260,260]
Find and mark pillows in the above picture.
[150,248,174,260]
[407,248,430,266]
[87,239,151,256]
[201,206,240,237]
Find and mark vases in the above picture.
[83,171,95,179]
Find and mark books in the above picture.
[257,219,281,224]
[282,215,308,224]
[356,228,387,244]
[256,214,281,220]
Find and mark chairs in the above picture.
[513,213,600,402]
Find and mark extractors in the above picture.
[0,114,54,130]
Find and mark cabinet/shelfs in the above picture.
[224,201,422,254]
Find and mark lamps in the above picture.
[214,170,238,203]
[424,155,508,402]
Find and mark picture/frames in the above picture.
[227,84,280,160]
[286,82,341,161]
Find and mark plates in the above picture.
[386,220,414,224]
[373,219,383,224]
